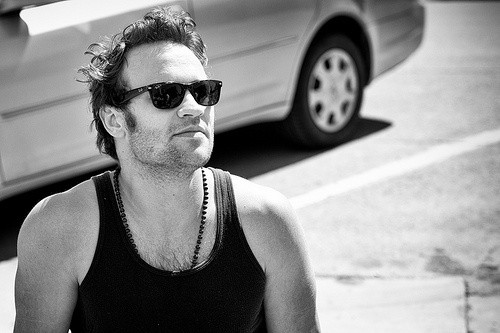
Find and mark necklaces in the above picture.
[109,166,210,276]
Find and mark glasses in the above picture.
[116,79,222,109]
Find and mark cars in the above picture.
[0,0,426,203]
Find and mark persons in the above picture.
[14,7,318,333]
